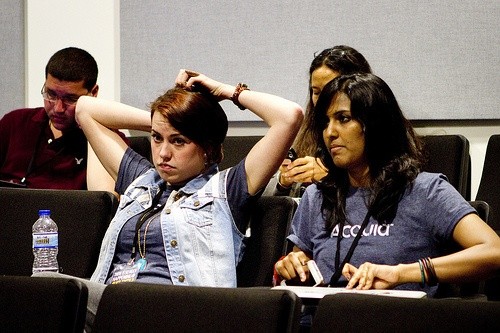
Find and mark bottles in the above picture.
[31,209,60,273]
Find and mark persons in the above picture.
[31,69,305,333]
[261,44,372,197]
[275,70,500,291]
[0,47,127,190]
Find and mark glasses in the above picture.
[313,48,352,58]
[40,82,92,106]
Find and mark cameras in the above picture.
[285,148,298,163]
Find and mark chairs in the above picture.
[0,134,500,333]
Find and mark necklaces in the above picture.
[133,211,162,271]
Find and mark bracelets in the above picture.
[417,257,440,288]
[231,82,252,109]
